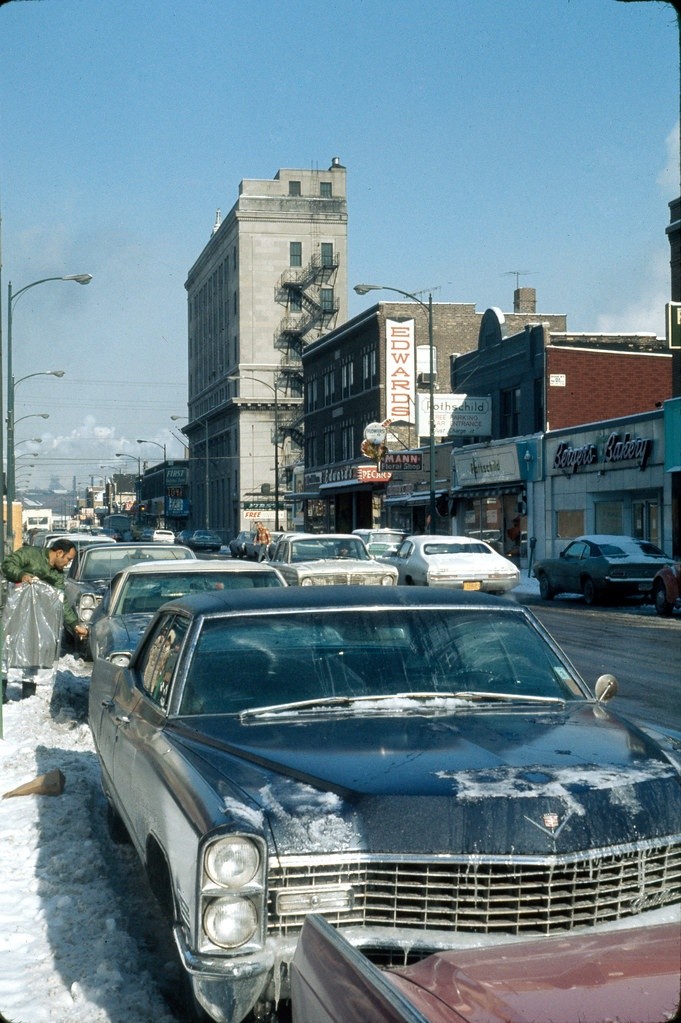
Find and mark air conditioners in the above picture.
[421,372,438,384]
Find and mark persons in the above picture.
[254,521,272,562]
[0,538,88,703]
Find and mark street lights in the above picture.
[88,474,106,516]
[170,414,209,530]
[226,376,279,533]
[115,454,140,525]
[100,466,121,514]
[5,272,94,592]
[353,283,436,535]
[137,439,167,529]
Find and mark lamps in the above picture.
[524,450,533,461]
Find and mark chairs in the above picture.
[133,596,170,609]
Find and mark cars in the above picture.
[87,560,345,669]
[87,584,681,1022]
[22,527,223,580]
[288,914,681,1023]
[228,531,329,561]
[351,528,405,559]
[260,533,399,588]
[463,527,528,558]
[652,563,681,619]
[63,542,225,662]
[376,534,521,596]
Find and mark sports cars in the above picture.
[532,535,681,606]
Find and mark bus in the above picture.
[103,514,131,540]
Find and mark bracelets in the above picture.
[74,625,81,629]
